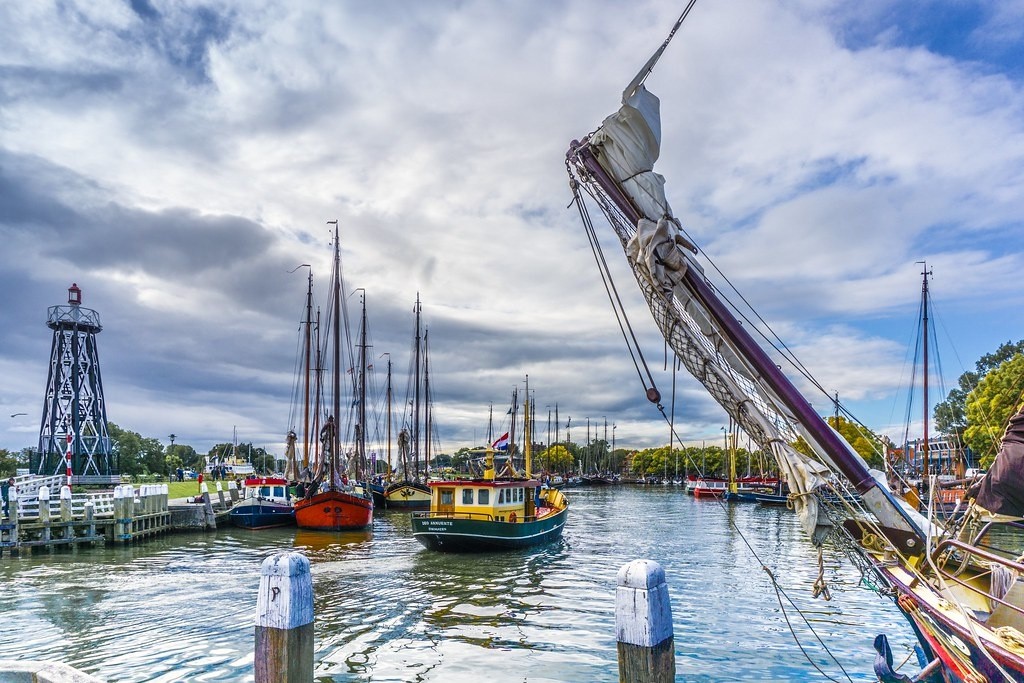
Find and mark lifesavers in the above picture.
[508,511,517,523]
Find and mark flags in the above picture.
[491,433,508,450]
[367,365,372,371]
[347,368,353,374]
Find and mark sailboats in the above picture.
[229,0,1024,683]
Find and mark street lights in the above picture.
[256,449,261,477]
[168,435,177,484]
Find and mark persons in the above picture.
[174,467,184,482]
[2,478,15,517]
[211,467,225,482]
[294,481,305,501]
[320,479,329,492]
